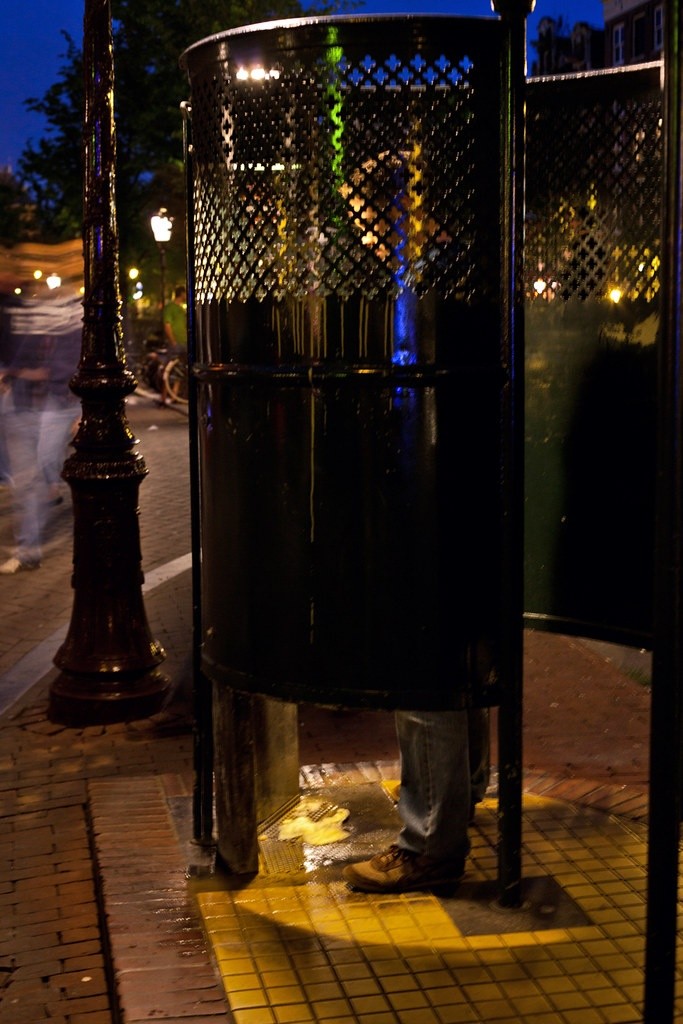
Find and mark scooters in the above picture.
[143,334,166,388]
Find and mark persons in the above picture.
[159,286,187,410]
[338,152,473,891]
[562,206,608,301]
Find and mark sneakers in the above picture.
[341,843,466,890]
[393,784,476,819]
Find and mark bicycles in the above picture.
[164,350,191,404]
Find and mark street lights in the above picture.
[152,207,173,348]
[129,265,139,350]
